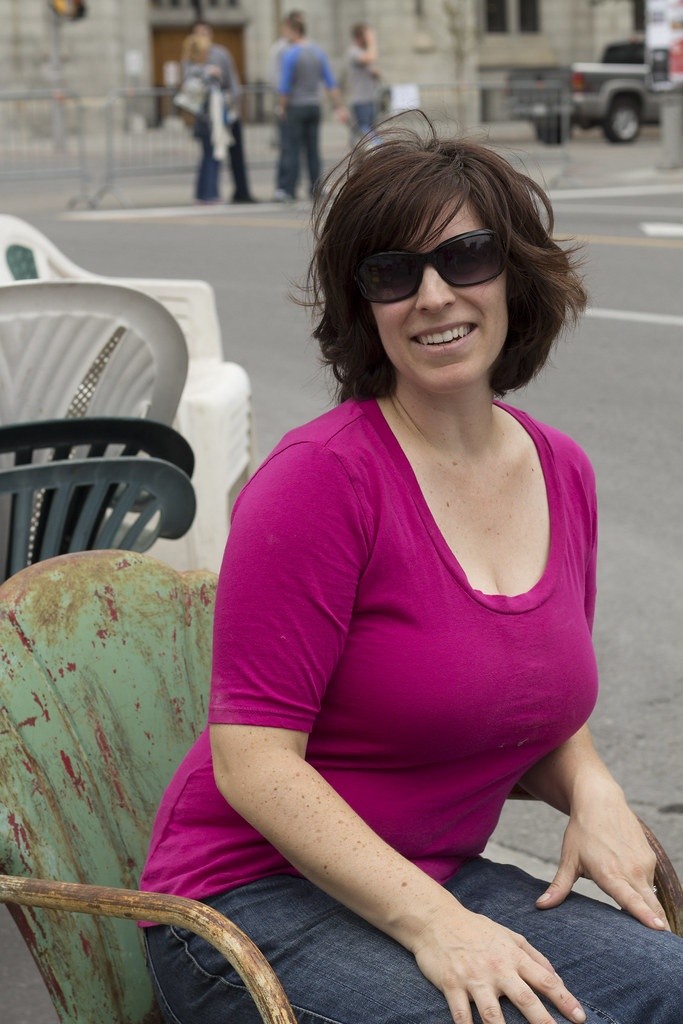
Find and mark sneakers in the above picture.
[270,190,295,202]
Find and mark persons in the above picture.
[138,109,683,1024]
[347,22,384,146]
[271,10,351,202]
[182,20,256,203]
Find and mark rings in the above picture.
[652,887,658,893]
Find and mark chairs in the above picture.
[0,548,682,1024]
[1,212,257,581]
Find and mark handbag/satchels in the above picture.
[172,76,210,116]
[373,79,391,111]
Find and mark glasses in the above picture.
[353,227,509,303]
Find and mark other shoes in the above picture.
[196,197,221,204]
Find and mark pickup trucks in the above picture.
[510,36,683,145]
